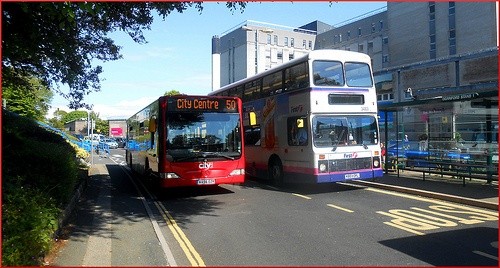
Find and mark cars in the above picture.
[88,132,124,149]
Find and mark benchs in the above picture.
[397,167,499,187]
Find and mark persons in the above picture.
[381,147,385,163]
[295,128,308,145]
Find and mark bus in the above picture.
[207,49,384,187]
[125,94,245,188]
[379,98,499,170]
